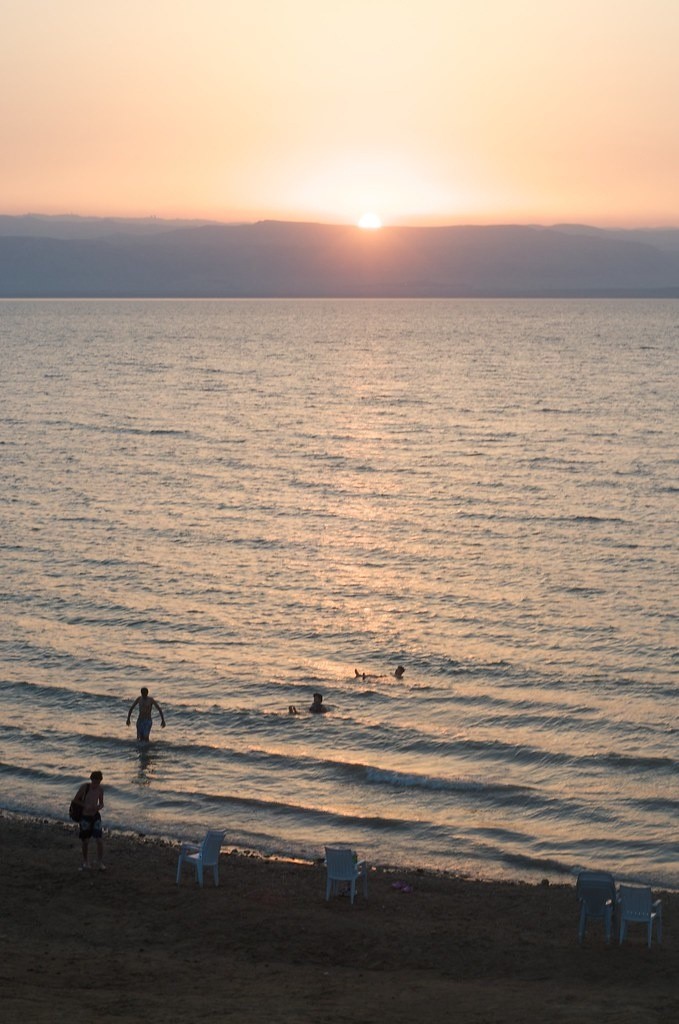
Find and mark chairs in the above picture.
[325,846,368,904]
[175,829,228,886]
[577,869,622,945]
[618,883,662,947]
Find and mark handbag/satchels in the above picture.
[70,783,90,823]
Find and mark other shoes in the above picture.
[83,861,92,869]
[95,863,107,871]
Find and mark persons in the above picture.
[355,665,405,680]
[126,687,166,742]
[289,692,327,714]
[69,771,107,870]
